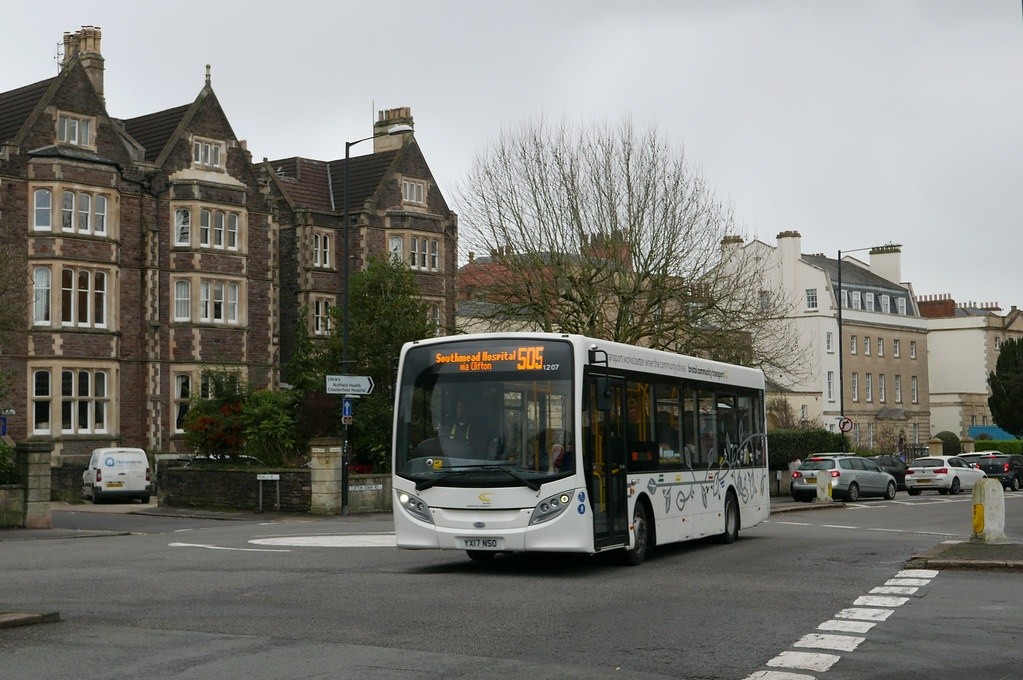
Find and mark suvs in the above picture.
[789,452,898,502]
[864,454,910,490]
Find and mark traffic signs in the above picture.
[326,375,375,394]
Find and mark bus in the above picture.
[392,332,770,565]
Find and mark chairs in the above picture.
[683,411,702,447]
[654,411,676,450]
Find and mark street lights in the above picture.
[839,244,903,454]
[341,125,416,517]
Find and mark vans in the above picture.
[80,448,152,504]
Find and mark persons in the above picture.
[628,407,638,422]
[445,398,475,444]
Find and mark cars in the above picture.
[904,454,989,496]
[346,455,373,474]
[976,453,1023,492]
[955,451,1004,471]
